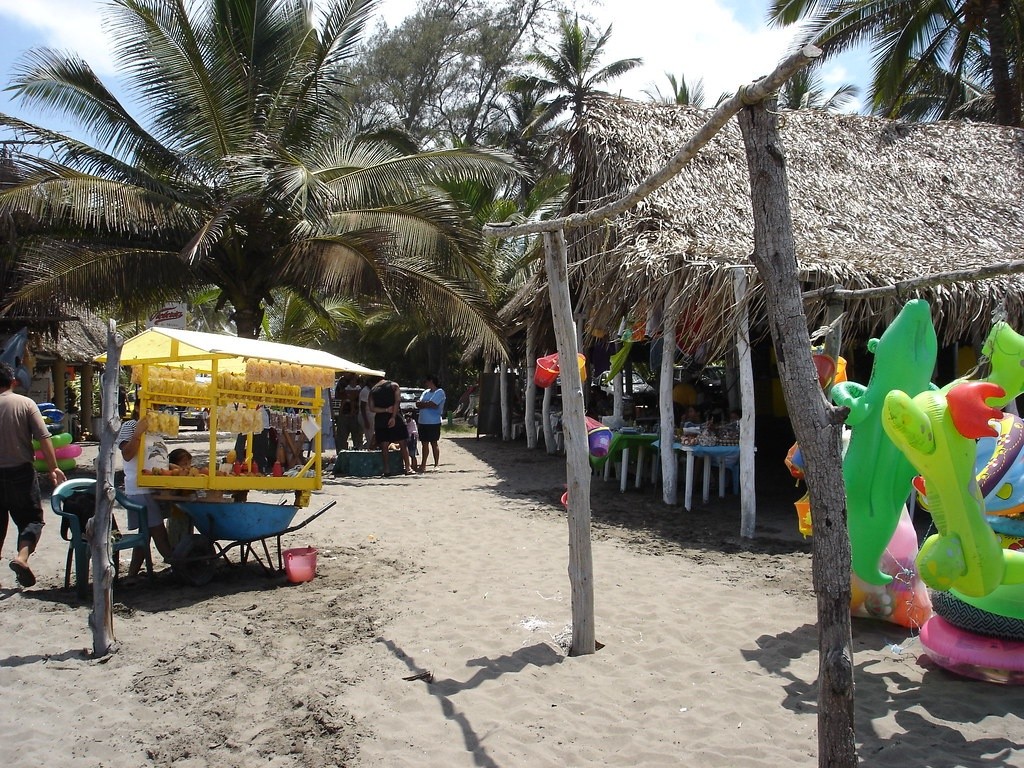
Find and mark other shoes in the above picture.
[432,466,440,471]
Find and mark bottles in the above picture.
[273,459,282,477]
[219,457,258,475]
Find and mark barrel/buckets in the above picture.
[578,353,587,382]
[588,426,613,457]
[834,356,847,383]
[812,353,837,402]
[561,491,567,510]
[282,546,319,584]
[534,353,560,388]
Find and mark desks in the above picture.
[652,439,759,510]
[602,429,658,493]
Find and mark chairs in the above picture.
[51,476,157,589]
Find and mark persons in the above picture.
[118,398,175,590]
[673,377,742,491]
[416,374,445,472]
[15,356,29,395]
[589,385,614,419]
[166,449,196,551]
[334,372,400,452]
[369,372,415,476]
[0,362,67,588]
[403,408,419,471]
[203,408,208,431]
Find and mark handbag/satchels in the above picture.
[340,399,356,417]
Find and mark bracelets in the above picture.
[385,408,386,412]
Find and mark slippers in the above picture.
[405,469,417,475]
[383,472,390,477]
[9,560,37,587]
